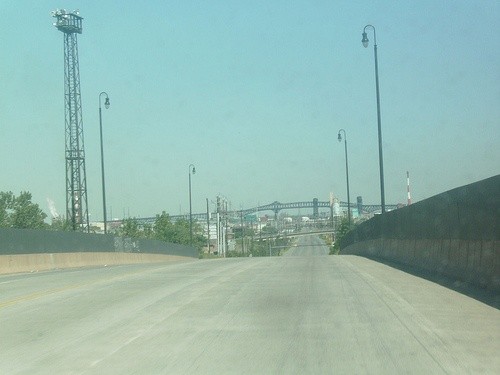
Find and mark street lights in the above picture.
[99,92,114,234]
[187,164,197,246]
[359,24,386,214]
[336,128,353,228]
